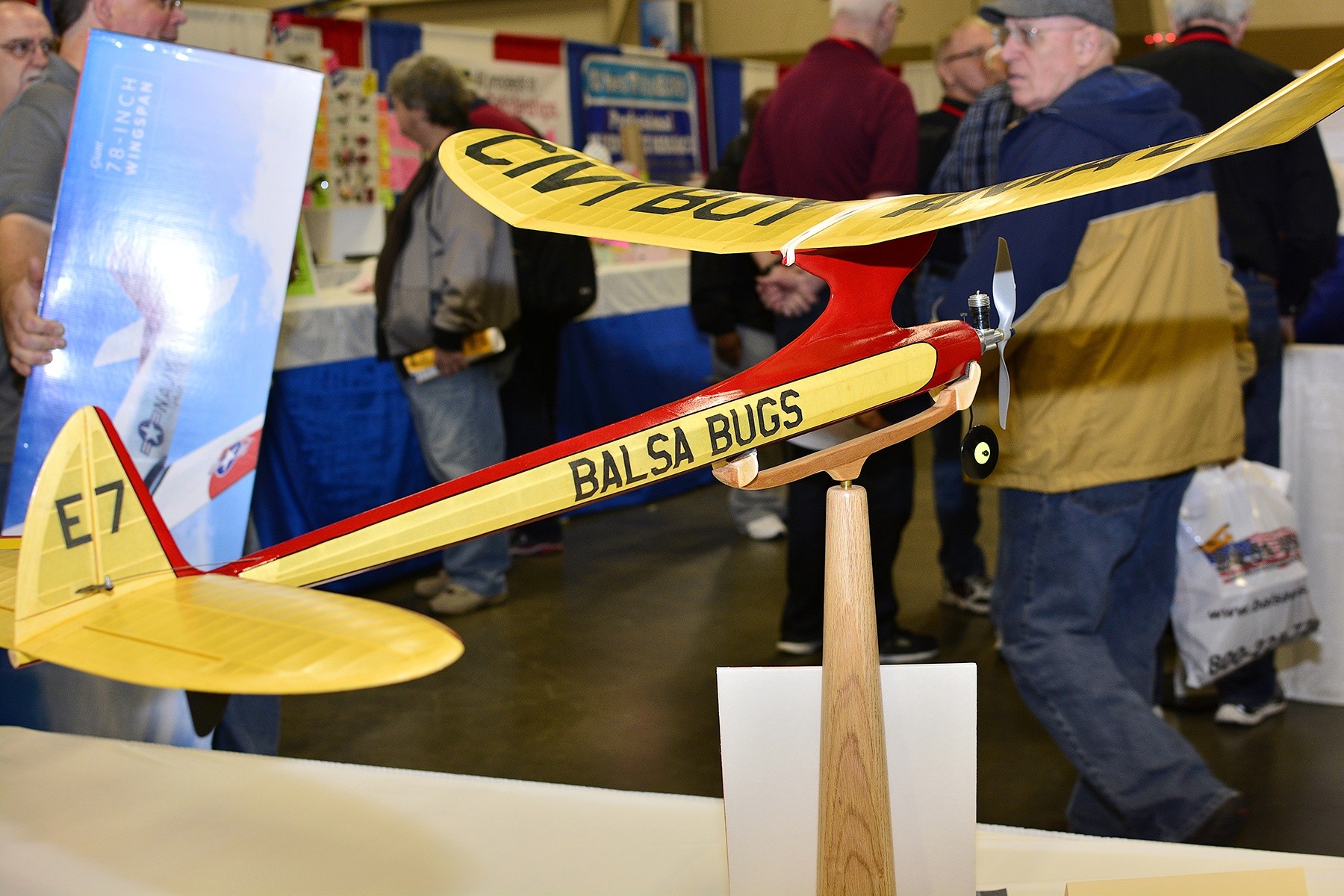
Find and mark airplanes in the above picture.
[2,47,1344,751]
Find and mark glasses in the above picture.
[997,25,1083,45]
[0,36,61,59]
[942,44,987,65]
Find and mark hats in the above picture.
[975,0,1121,37]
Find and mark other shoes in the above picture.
[879,623,941,664]
[507,532,566,556]
[735,514,789,542]
[774,622,824,657]
[1211,681,1287,728]
[935,574,995,615]
[414,567,509,617]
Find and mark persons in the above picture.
[372,52,567,619]
[915,2,1344,846]
[0,1,284,755]
[0,0,53,113]
[689,0,944,664]
[927,2,1262,846]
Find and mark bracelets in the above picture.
[760,258,783,275]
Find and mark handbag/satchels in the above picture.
[1166,456,1319,687]
